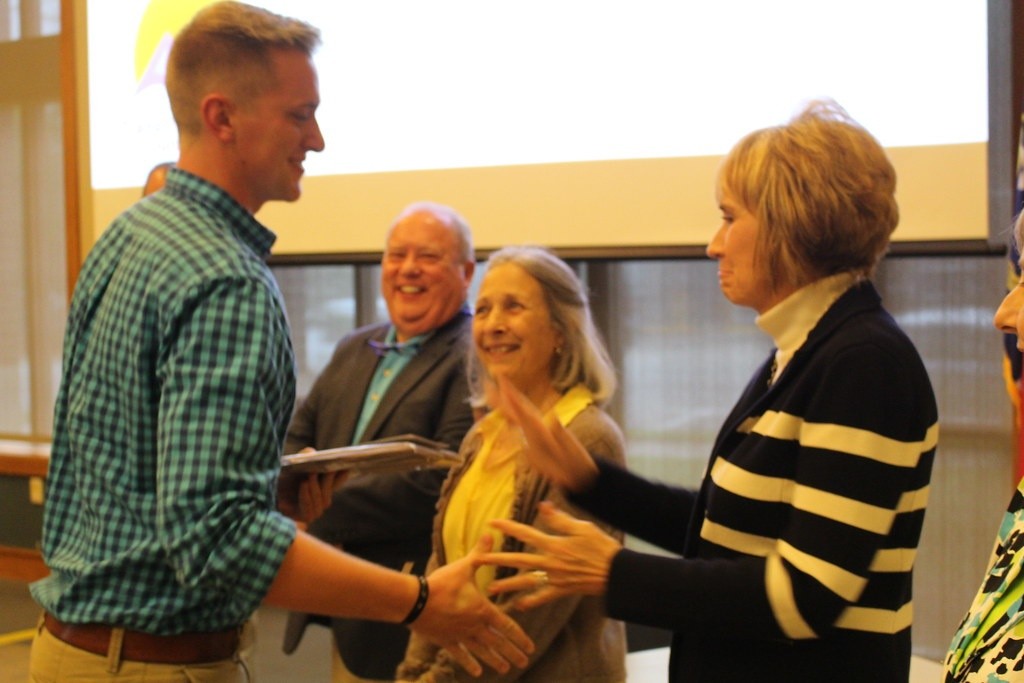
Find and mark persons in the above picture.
[396,246,628,683]
[941,207,1024,683]
[28,0,535,683]
[478,99,939,683]
[277,200,474,683]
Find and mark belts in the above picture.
[43,610,239,664]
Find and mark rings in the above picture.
[534,571,547,585]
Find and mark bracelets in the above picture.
[400,575,429,626]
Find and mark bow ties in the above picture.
[368,338,408,357]
